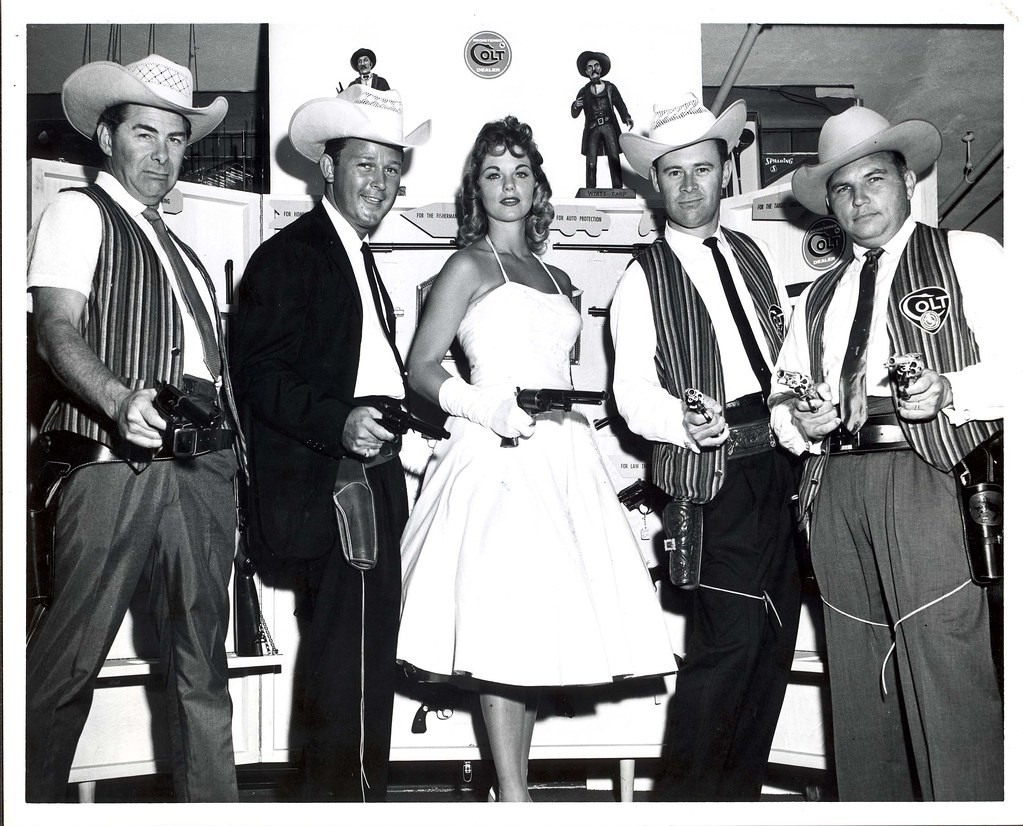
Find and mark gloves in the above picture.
[438,375,535,441]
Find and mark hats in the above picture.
[618,92,746,180]
[792,106,943,216]
[288,84,432,165]
[62,54,229,146]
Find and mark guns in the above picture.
[777,368,843,435]
[373,401,451,457]
[616,477,676,517]
[685,387,727,449]
[152,380,219,432]
[500,386,610,449]
[887,352,924,400]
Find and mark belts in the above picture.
[830,415,913,455]
[165,427,235,459]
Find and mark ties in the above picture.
[140,207,220,383]
[702,236,773,402]
[362,242,411,416]
[838,247,885,435]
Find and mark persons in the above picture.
[766,105,1004,802]
[570,50,636,189]
[27,51,251,803]
[396,115,679,803]
[608,92,811,803]
[335,47,391,95]
[222,80,433,802]
